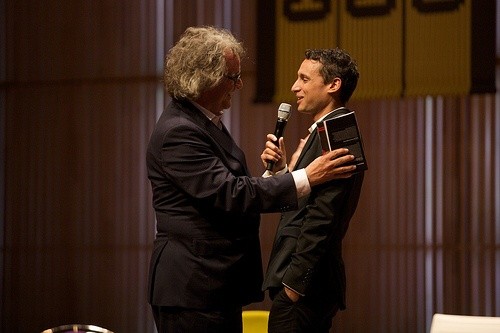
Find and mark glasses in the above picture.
[224,72,243,80]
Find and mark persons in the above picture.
[144,25,358,333]
[257,47,367,333]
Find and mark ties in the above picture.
[287,133,311,174]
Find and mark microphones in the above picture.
[266,103,292,170]
